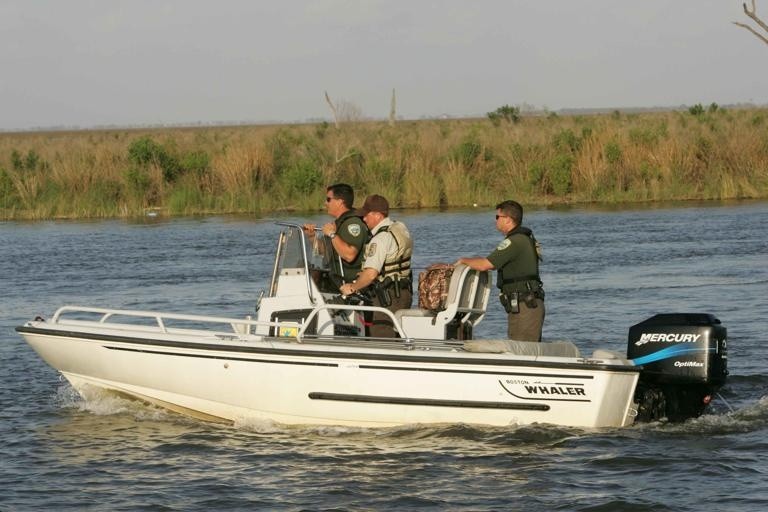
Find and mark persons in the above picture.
[339,195,413,341]
[302,184,372,336]
[452,200,545,342]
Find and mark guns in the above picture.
[378,277,392,307]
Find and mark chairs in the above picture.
[395,262,493,339]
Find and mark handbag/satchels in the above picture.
[417,262,455,326]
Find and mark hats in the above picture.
[354,194,389,217]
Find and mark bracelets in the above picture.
[350,283,356,293]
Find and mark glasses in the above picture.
[496,215,506,219]
[327,197,336,202]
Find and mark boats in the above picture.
[14,220,730,439]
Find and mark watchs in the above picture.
[330,233,337,240]
